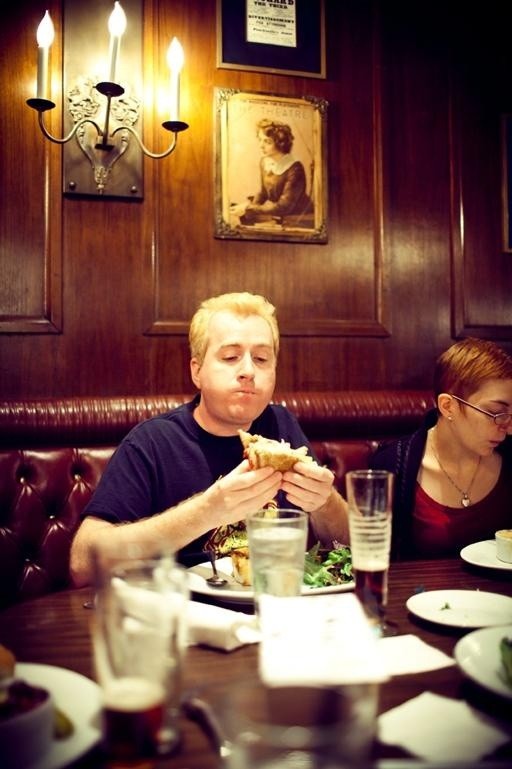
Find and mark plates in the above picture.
[406,589,512,629]
[6,662,103,767]
[453,627,512,696]
[188,555,357,604]
[460,538,511,570]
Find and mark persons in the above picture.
[230,120,312,226]
[71,292,361,587]
[359,338,512,562]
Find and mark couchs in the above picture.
[1,386,443,608]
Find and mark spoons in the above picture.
[207,548,227,588]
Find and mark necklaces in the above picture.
[428,439,482,507]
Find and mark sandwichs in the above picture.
[236,427,315,472]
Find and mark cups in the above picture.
[1,686,53,764]
[246,508,309,614]
[347,468,392,571]
[495,530,511,565]
[228,675,381,766]
[85,557,187,712]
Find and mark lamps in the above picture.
[23,1,189,201]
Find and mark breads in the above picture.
[232,547,250,585]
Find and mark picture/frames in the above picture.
[210,88,333,245]
[215,0,330,81]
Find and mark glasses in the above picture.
[452,394,512,425]
